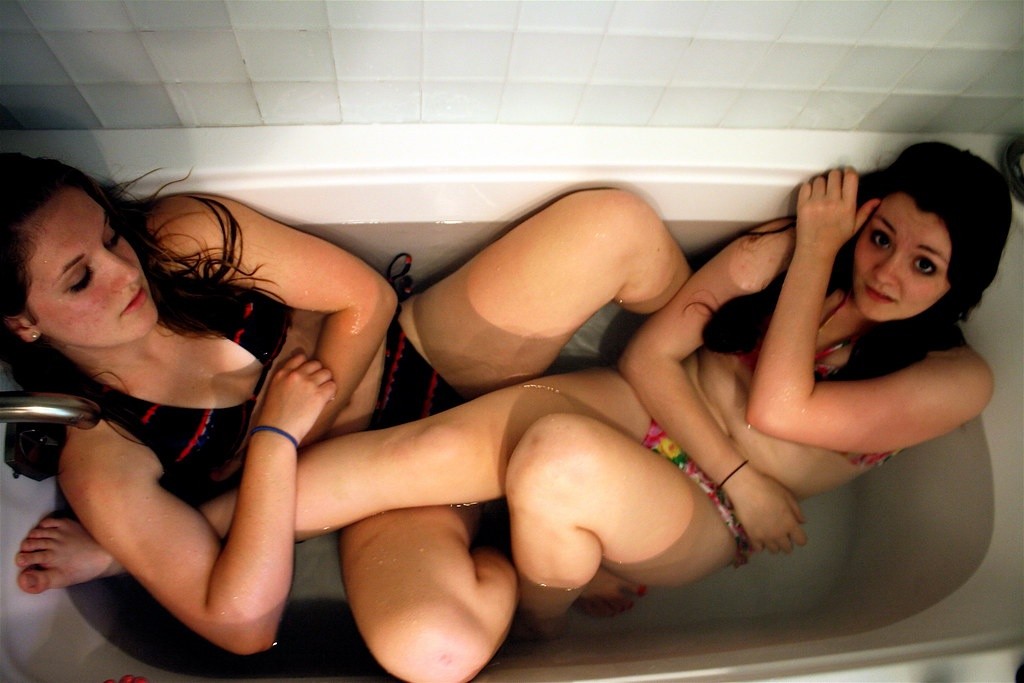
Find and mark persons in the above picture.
[0,141,691,683]
[13,140,1013,644]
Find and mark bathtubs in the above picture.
[0,160,1023,683]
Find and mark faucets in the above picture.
[0,390,104,431]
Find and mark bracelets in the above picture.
[248,426,299,452]
[716,458,751,490]
